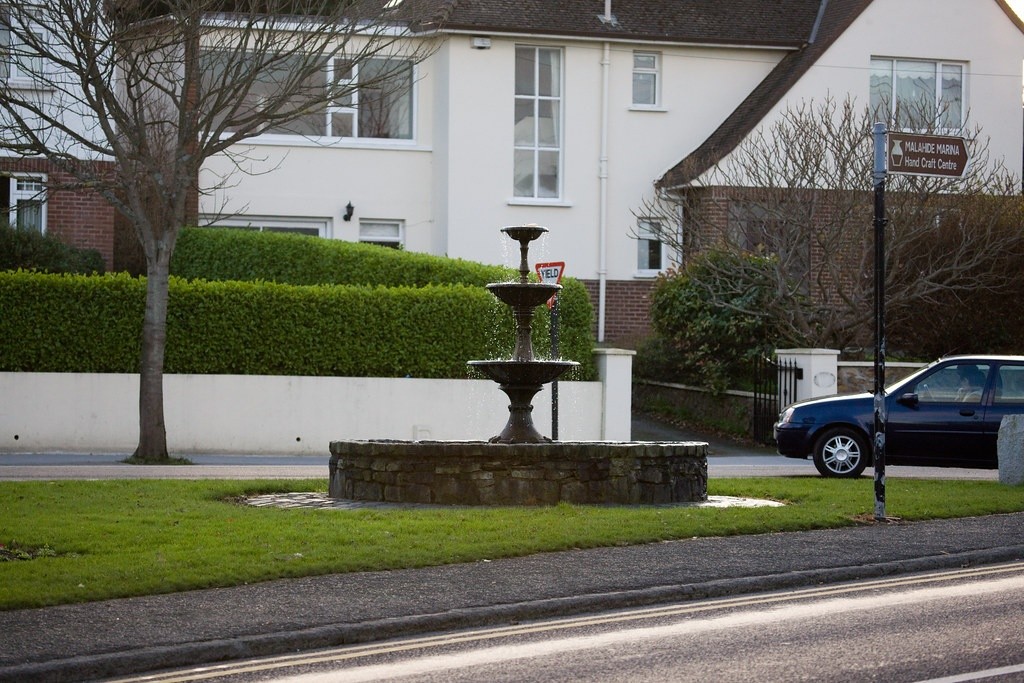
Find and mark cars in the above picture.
[773,354,1024,480]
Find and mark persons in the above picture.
[956,364,985,402]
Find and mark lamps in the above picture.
[343,201,354,221]
